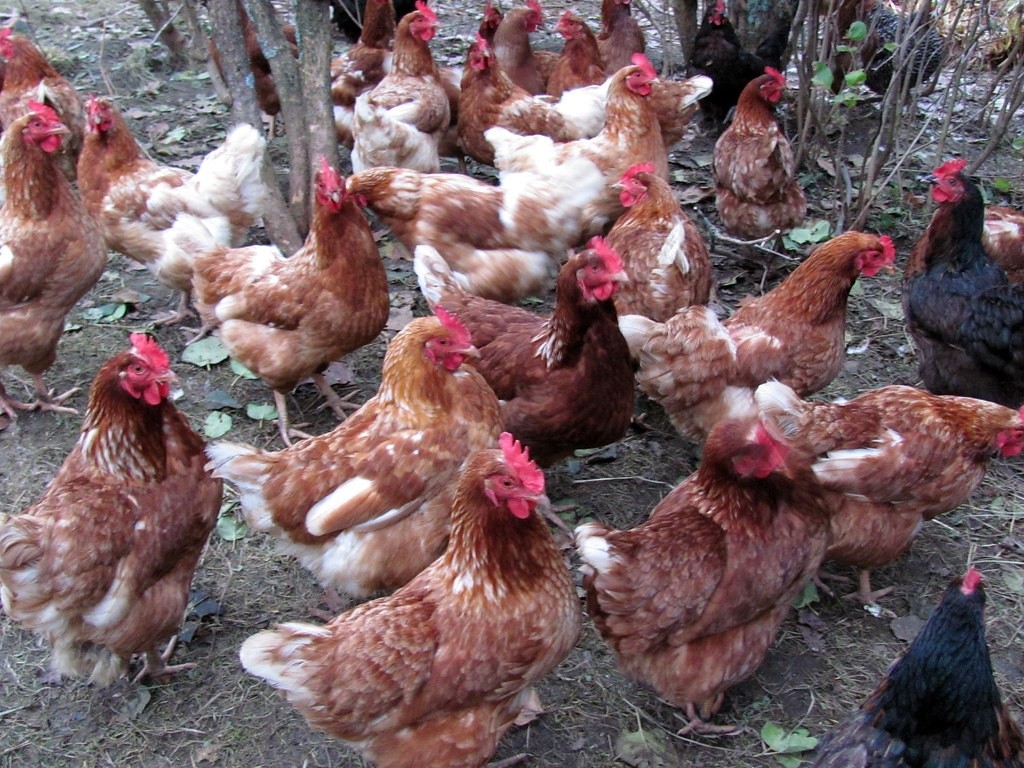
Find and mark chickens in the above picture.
[0,1,1023,768]
